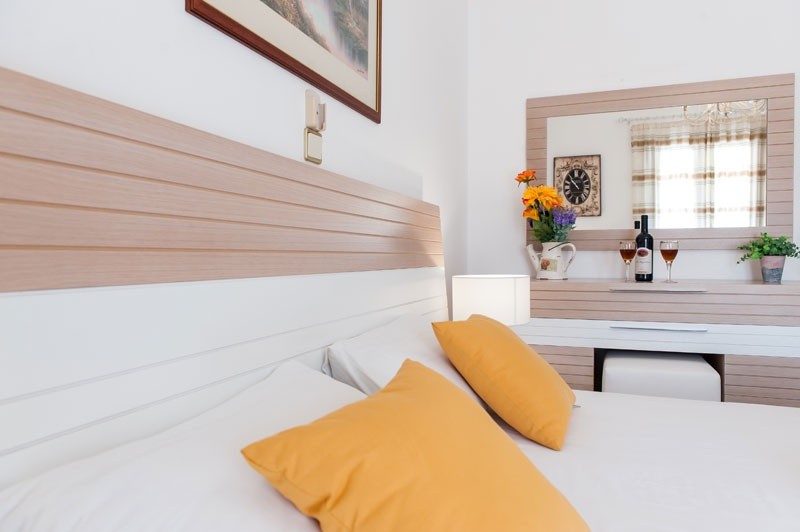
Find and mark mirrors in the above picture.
[526,73,796,251]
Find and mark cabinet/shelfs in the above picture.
[508,281,800,404]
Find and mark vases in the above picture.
[526,243,577,280]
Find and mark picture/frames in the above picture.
[185,0,381,124]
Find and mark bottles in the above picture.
[635,215,653,282]
[634,221,640,230]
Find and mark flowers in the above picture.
[515,170,577,242]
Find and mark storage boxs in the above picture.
[452,275,530,326]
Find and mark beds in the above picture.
[0,59,800,532]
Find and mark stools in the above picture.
[601,350,722,402]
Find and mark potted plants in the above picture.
[736,233,800,284]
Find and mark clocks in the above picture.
[554,154,601,216]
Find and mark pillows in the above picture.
[0,313,590,532]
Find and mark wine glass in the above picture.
[659,240,679,283]
[620,241,635,283]
